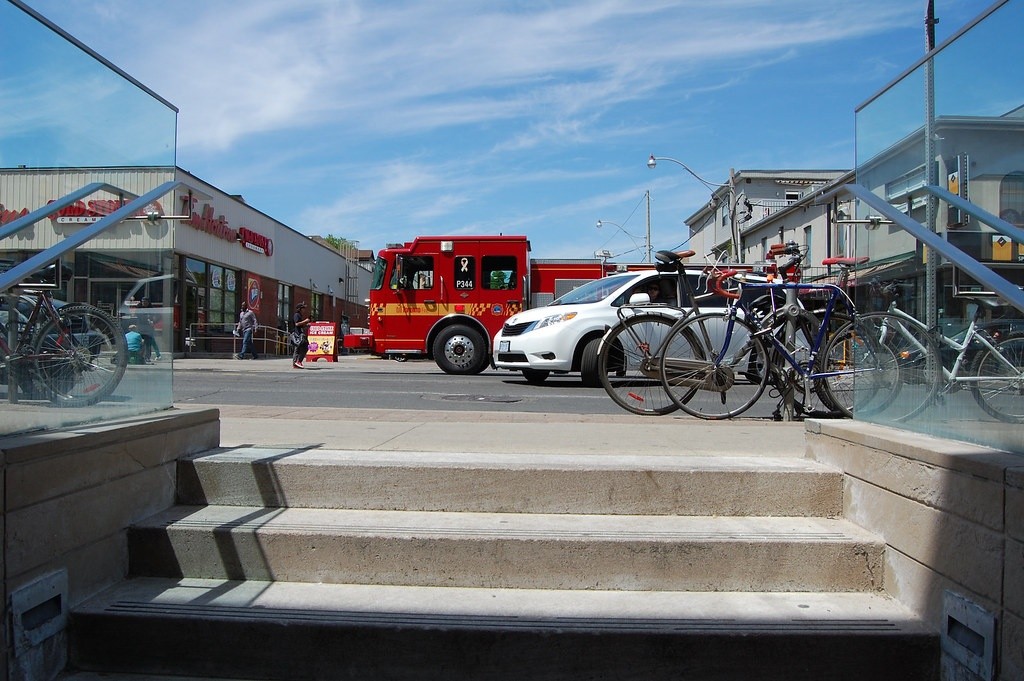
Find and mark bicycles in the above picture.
[0,264,129,407]
[596,239,942,422]
[829,264,1024,423]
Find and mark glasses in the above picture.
[648,287,659,291]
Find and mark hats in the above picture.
[142,297,149,301]
[296,303,307,310]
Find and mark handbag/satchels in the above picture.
[290,332,306,348]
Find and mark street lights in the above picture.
[647,155,740,262]
[596,219,651,262]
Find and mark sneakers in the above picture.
[295,361,304,368]
[292,366,298,368]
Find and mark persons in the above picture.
[646,282,667,303]
[136,296,154,365]
[122,324,146,365]
[235,301,259,360]
[291,301,310,369]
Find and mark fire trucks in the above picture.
[369,232,778,375]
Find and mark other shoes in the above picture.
[144,358,151,362]
[253,355,259,359]
[234,354,243,360]
[156,355,161,360]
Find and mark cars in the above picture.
[493,270,821,387]
[0,290,102,376]
[893,318,1024,384]
